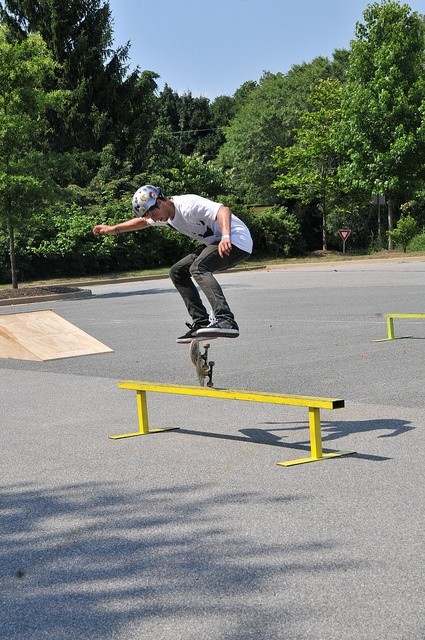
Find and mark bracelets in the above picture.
[219,235,231,240]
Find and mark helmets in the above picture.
[132,185,161,218]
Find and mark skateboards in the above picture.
[190,339,215,387]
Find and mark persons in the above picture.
[93,184,255,343]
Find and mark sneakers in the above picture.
[176,320,217,343]
[197,310,240,338]
